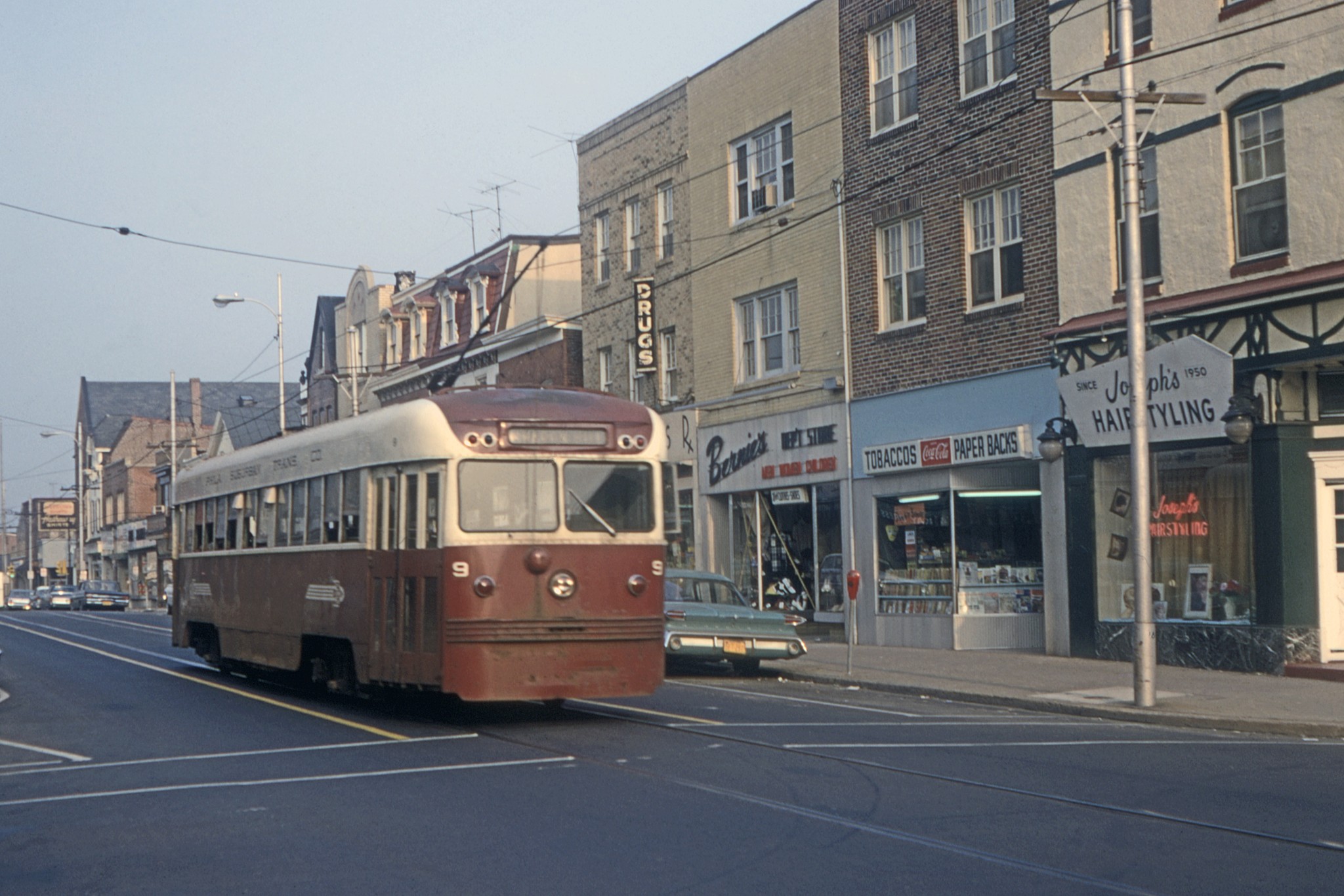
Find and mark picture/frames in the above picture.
[1107,487,1132,519]
[1105,531,1128,563]
[1182,561,1216,624]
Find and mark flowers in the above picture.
[1207,578,1252,607]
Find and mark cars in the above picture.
[660,568,807,676]
[6,585,76,610]
[163,584,173,616]
[819,551,845,595]
[69,579,129,611]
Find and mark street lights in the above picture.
[41,421,86,585]
[212,273,284,436]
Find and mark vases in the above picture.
[1221,595,1240,621]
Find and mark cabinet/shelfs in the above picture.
[880,579,954,615]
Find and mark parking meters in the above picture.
[844,570,861,675]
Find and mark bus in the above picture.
[0,572,13,609]
[172,235,667,713]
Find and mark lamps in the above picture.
[1034,416,1081,468]
[1220,390,1263,446]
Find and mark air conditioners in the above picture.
[751,183,778,213]
